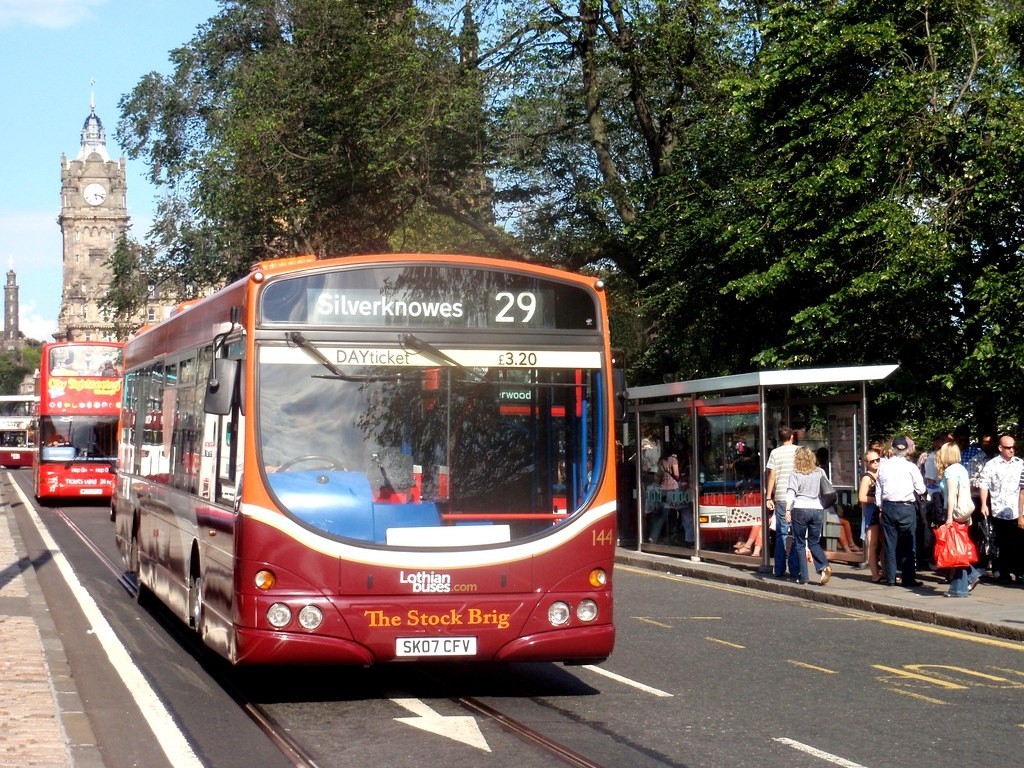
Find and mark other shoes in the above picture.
[902,578,923,588]
[796,578,808,585]
[967,578,980,592]
[786,574,800,583]
[648,538,655,544]
[775,572,790,580]
[944,592,961,597]
[821,567,831,584]
[733,540,746,549]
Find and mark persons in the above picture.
[48,426,72,447]
[558,421,708,548]
[65,358,120,376]
[731,427,862,585]
[264,405,349,472]
[8,436,19,445]
[859,426,1024,597]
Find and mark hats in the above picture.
[892,438,907,450]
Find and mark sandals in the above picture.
[849,544,863,552]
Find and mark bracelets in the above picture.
[766,498,772,501]
[787,510,790,511]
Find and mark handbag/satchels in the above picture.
[978,514,999,558]
[932,521,979,568]
[950,466,975,522]
[818,469,836,509]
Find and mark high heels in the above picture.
[734,546,752,556]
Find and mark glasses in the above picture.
[867,458,880,464]
[1002,446,1016,449]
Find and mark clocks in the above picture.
[84,183,107,207]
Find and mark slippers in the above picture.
[871,576,889,584]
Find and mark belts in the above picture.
[884,500,911,506]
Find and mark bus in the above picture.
[0,393,36,469]
[27,341,130,505]
[607,395,768,546]
[108,254,631,672]
